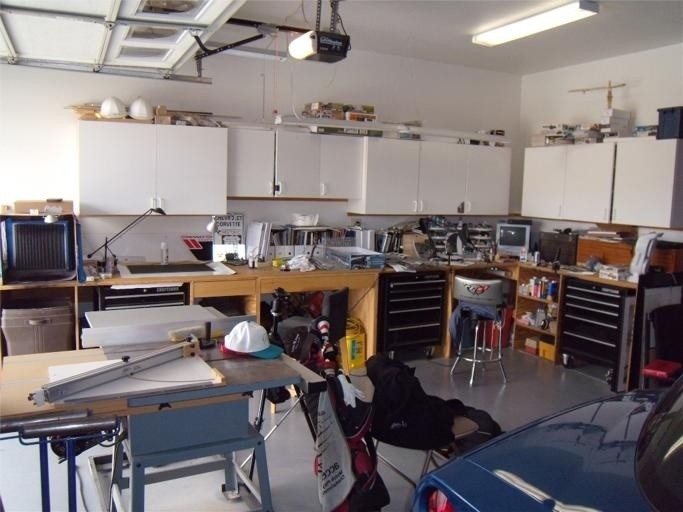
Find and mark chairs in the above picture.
[276,287,348,365]
[374,416,479,489]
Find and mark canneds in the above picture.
[534,251,540,263]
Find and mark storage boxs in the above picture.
[656,106,682,140]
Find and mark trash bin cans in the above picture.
[277,316,317,364]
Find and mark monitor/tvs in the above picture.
[496,223,533,257]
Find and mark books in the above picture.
[246,222,272,263]
[272,230,329,245]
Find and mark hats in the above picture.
[221,320,284,359]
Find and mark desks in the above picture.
[0,260,378,359]
[0,336,328,512]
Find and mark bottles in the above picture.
[248,252,258,270]
[160,243,169,266]
[43,199,64,215]
[518,275,558,329]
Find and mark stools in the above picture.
[450,274,507,386]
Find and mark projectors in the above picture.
[288,31,351,64]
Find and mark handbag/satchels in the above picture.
[365,355,446,448]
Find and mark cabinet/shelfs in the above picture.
[347,137,466,215]
[521,144,567,220]
[228,126,320,198]
[429,227,493,261]
[73,120,228,217]
[576,234,683,273]
[564,139,681,229]
[466,144,512,215]
[512,264,562,366]
[320,134,364,199]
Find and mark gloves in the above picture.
[337,373,365,409]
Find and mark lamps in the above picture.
[87,208,166,277]
[472,1,600,47]
[207,215,219,233]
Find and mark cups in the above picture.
[520,246,527,264]
[272,258,283,268]
[561,352,572,368]
